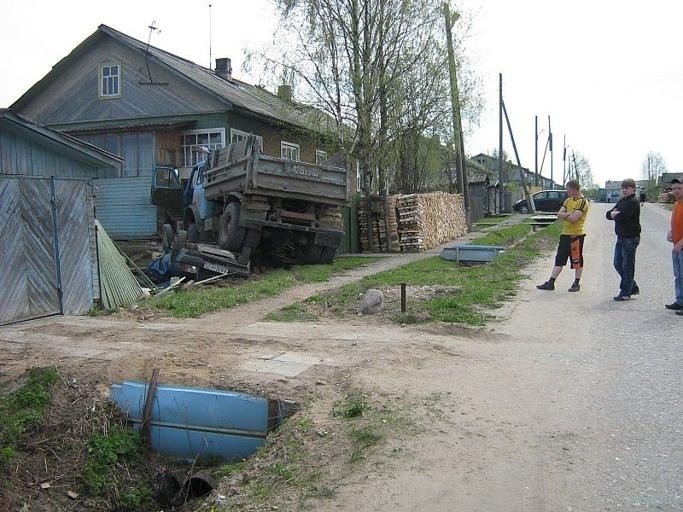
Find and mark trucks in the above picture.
[149,138,360,264]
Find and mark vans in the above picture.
[512,189,571,214]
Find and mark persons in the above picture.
[606,179,641,301]
[536,180,589,292]
[640,188,646,206]
[665,178,683,314]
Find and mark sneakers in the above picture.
[568,283,580,292]
[630,286,640,295]
[614,294,630,301]
[537,282,554,290]
[676,311,683,315]
[665,303,683,310]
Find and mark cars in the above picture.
[148,229,251,283]
